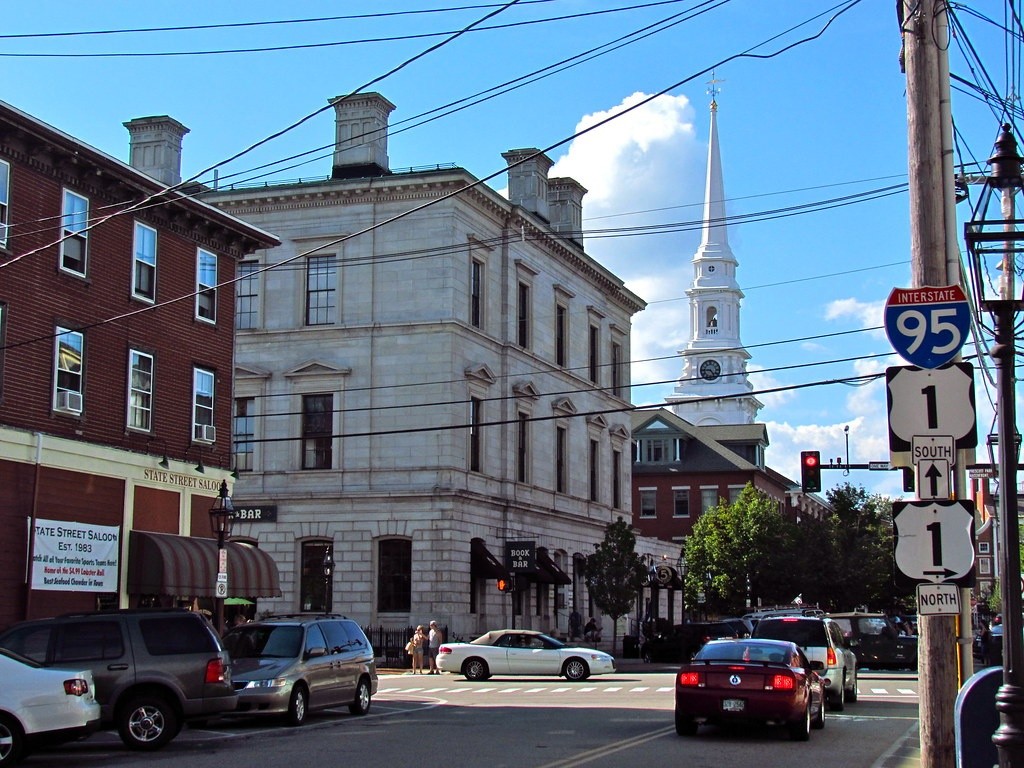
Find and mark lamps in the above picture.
[150,436,169,469]
[185,445,205,474]
[219,452,239,479]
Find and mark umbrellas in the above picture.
[223,597,254,606]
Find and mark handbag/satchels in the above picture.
[405,642,414,654]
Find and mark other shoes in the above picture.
[412,672,423,675]
[428,670,440,675]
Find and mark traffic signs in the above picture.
[868,461,898,471]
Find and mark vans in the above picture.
[220,613,379,724]
[741,609,826,628]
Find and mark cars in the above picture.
[674,636,827,742]
[0,648,102,768]
[435,629,616,682]
[973,624,1003,667]
[722,619,753,638]
[891,614,919,672]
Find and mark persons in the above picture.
[229,615,256,658]
[890,616,918,671]
[550,606,602,645]
[410,621,442,674]
[976,616,1003,668]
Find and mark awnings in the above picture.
[127,532,282,598]
[472,541,570,584]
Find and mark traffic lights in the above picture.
[801,451,820,492]
[995,615,1002,624]
[497,576,511,592]
[516,577,529,591]
[902,466,914,492]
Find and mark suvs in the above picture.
[640,621,739,664]
[0,606,240,750]
[750,613,858,711]
[821,613,898,672]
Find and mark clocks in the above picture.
[700,360,721,380]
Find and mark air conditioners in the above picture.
[195,425,216,442]
[0,222,7,245]
[57,392,83,413]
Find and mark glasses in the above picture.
[417,629,421,632]
[429,624,434,627]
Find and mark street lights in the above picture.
[322,545,336,615]
[208,479,236,630]
